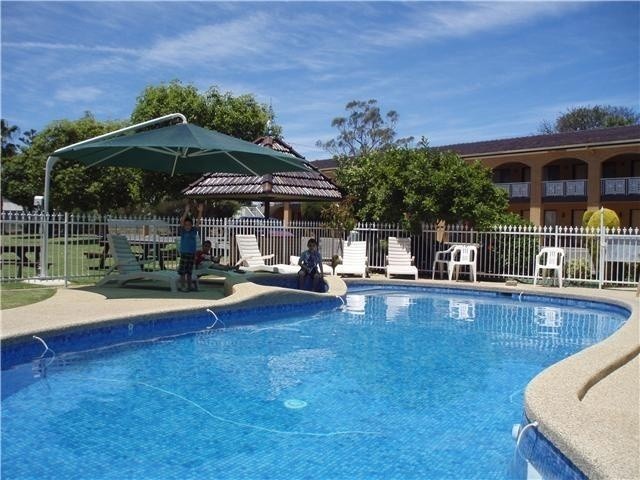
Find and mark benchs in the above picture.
[0,252,180,278]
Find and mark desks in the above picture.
[100,240,174,270]
[0,245,42,276]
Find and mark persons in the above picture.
[297,238,324,292]
[176,203,204,292]
[196,239,240,272]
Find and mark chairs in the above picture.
[534,247,565,288]
[236,235,299,274]
[384,237,418,280]
[174,239,256,289]
[96,234,200,292]
[288,254,332,275]
[431,245,477,282]
[345,294,563,328]
[334,240,367,277]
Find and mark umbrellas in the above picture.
[39,113,313,280]
